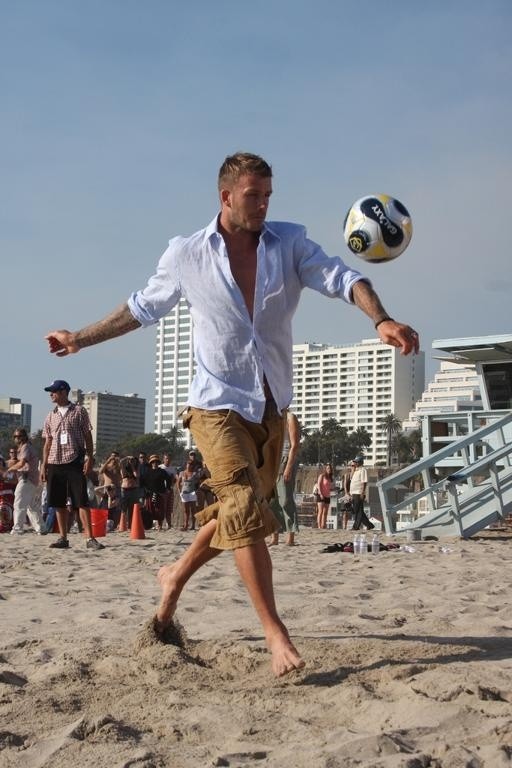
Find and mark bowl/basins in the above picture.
[407,530,421,541]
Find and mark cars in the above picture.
[299,458,347,467]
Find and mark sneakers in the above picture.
[87,538,105,549]
[50,540,69,548]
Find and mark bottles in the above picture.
[353,532,380,555]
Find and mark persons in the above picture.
[267,454,374,531]
[42,149,422,678]
[266,407,302,550]
[0,448,218,531]
[38,378,106,551]
[9,427,50,535]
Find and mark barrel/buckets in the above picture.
[87,507,108,538]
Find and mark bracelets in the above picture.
[374,315,394,331]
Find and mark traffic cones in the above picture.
[117,509,128,534]
[128,502,146,537]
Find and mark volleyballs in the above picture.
[344,195,412,263]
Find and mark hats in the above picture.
[149,455,161,463]
[45,380,70,392]
[353,455,362,462]
